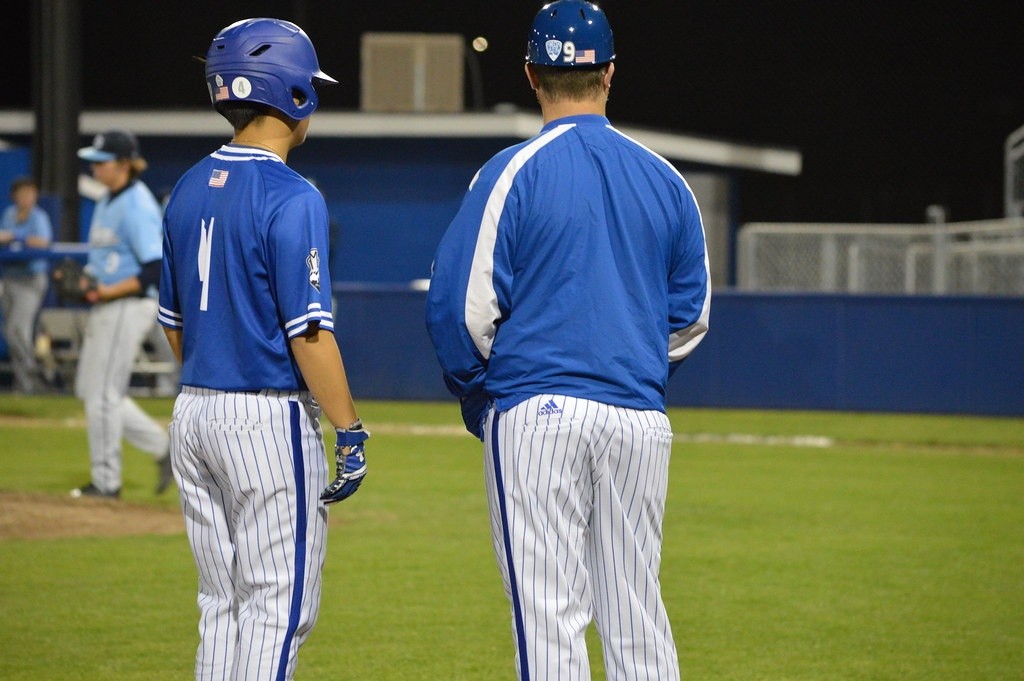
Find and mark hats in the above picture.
[77,129,136,163]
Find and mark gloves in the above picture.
[318,418,371,506]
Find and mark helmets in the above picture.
[526,0,616,68]
[205,18,339,120]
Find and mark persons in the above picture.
[144,19,367,681]
[0,180,53,391]
[50,131,172,495]
[423,0,713,681]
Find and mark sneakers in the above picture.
[154,451,172,496]
[69,483,123,500]
